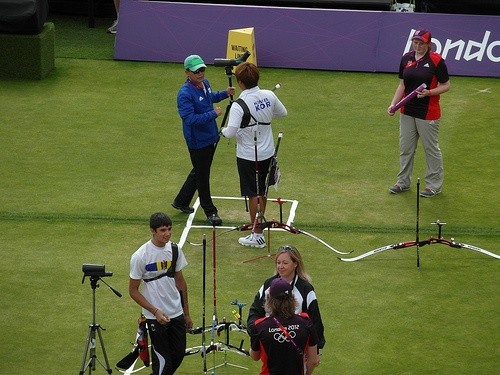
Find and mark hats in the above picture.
[184,54,207,72]
[268,278,292,297]
[411,30,431,43]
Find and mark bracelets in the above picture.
[153,308,159,316]
[428,89,432,96]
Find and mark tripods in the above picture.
[79,277,122,375]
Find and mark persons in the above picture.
[221,62,288,248]
[386,29,451,198]
[170,55,236,226]
[248,278,318,375]
[247,245,326,368]
[129,212,193,375]
[107,0,120,34]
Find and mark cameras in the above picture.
[214,51,251,66]
[82,264,113,276]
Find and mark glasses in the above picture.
[278,246,293,251]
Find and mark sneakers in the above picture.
[208,212,222,226]
[419,186,442,198]
[389,183,411,194]
[238,232,266,248]
[171,200,194,213]
